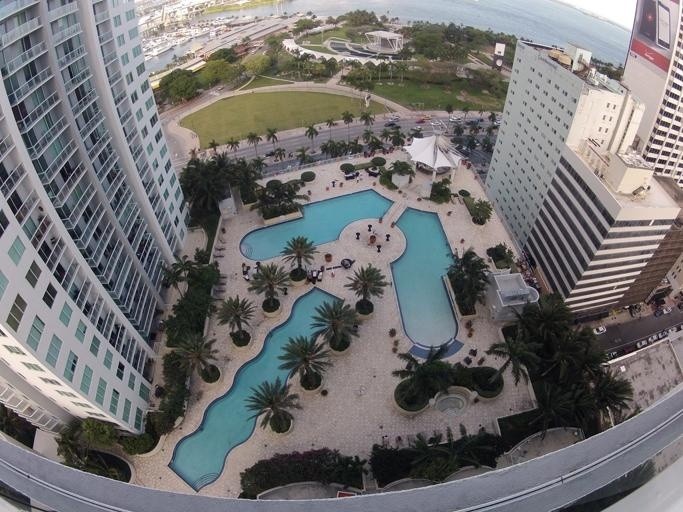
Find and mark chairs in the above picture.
[381,429,445,449]
[450,194,463,205]
[209,237,227,300]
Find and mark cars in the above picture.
[466,117,485,126]
[611,327,677,359]
[656,308,672,316]
[449,117,461,122]
[384,122,401,130]
[416,119,425,124]
[593,326,606,336]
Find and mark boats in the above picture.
[143,26,227,56]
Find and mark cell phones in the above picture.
[656,0,670,51]
[640,0,656,43]
